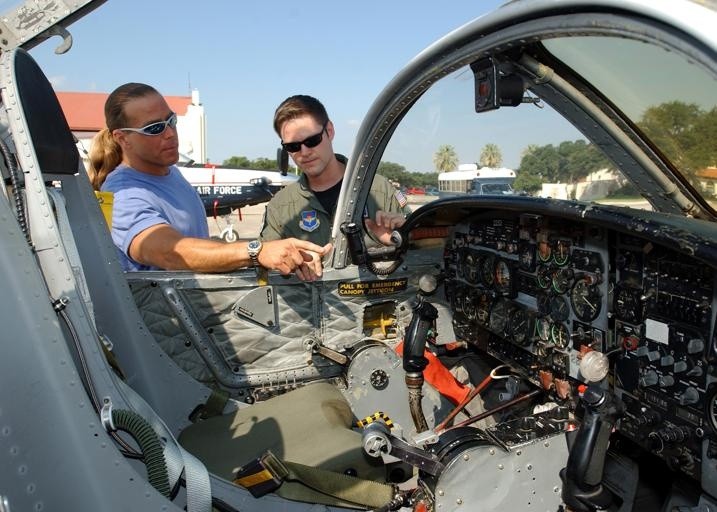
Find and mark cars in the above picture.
[400,187,439,196]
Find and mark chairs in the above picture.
[16,187,384,502]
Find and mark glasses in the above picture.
[281,124,327,153]
[120,112,178,136]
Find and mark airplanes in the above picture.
[76,139,301,244]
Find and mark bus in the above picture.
[438,164,516,199]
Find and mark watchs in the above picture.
[247,240,262,267]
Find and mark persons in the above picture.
[256,95,412,281]
[87,83,326,276]
